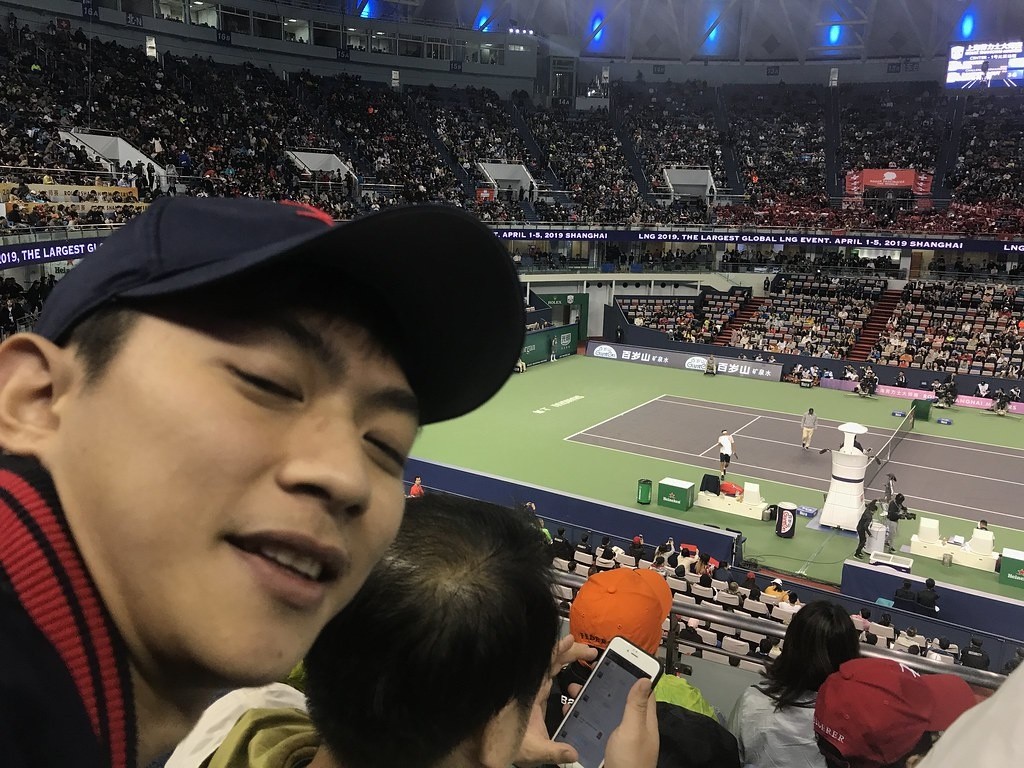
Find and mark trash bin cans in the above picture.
[636,478,654,505]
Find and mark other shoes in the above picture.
[802,442,805,449]
[724,469,727,475]
[889,548,897,554]
[853,551,864,561]
[721,475,724,480]
[805,447,810,451]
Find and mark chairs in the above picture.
[970,528,994,555]
[743,482,765,505]
[699,474,721,495]
[544,544,971,699]
[918,517,942,543]
[612,272,1024,386]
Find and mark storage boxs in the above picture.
[938,418,952,424]
[892,410,906,417]
[796,505,818,517]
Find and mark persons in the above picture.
[976,519,988,530]
[0,194,526,768]
[802,408,818,451]
[410,474,1023,768]
[716,429,736,480]
[549,332,557,362]
[2,11,1024,415]
[704,354,716,376]
[163,496,660,768]
[884,493,907,552]
[854,497,877,560]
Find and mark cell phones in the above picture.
[551,635,664,768]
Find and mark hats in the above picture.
[812,657,978,768]
[971,634,984,647]
[32,192,525,428]
[770,579,783,587]
[747,572,756,579]
[567,568,672,669]
[557,527,566,533]
[633,536,640,544]
[655,557,665,564]
[1016,646,1024,657]
[568,561,577,570]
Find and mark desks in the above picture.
[657,477,696,512]
[999,547,1024,589]
